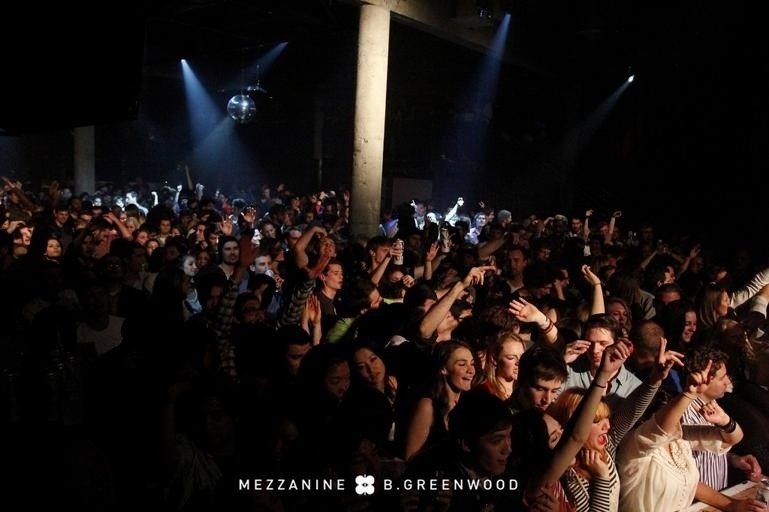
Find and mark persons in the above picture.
[0,164,769,512]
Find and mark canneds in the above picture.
[393,240,404,266]
[441,227,451,253]
